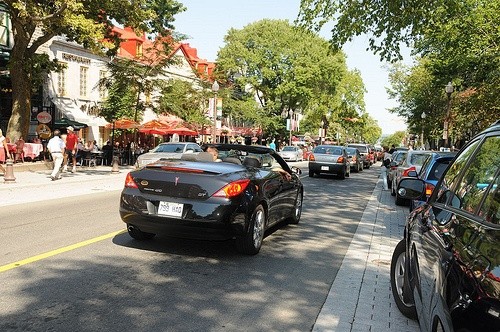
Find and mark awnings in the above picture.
[51,98,90,121]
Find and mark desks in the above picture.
[23,143,43,162]
[90,151,103,167]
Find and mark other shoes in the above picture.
[51,176,55,180]
[71,169,76,173]
[55,177,62,179]
[61,170,67,172]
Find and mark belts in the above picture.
[52,152,61,153]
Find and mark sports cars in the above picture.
[119,143,304,256]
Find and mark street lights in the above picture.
[445,81,454,147]
[421,112,426,150]
[321,120,324,145]
[289,108,293,146]
[212,80,219,143]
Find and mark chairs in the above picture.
[181,152,260,166]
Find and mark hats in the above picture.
[66,126,74,132]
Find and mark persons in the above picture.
[76,138,112,166]
[207,145,218,160]
[378,145,397,191]
[47,129,65,180]
[62,126,78,173]
[0,128,10,176]
[13,136,25,163]
[266,139,276,150]
[113,138,154,166]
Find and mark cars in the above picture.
[389,150,440,206]
[407,150,458,213]
[134,142,204,171]
[371,146,383,164]
[298,145,311,161]
[386,147,409,190]
[278,146,304,162]
[345,147,364,173]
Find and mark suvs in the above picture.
[389,118,500,332]
[308,144,352,179]
[348,144,374,169]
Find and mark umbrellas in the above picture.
[224,127,315,142]
[106,118,232,142]
